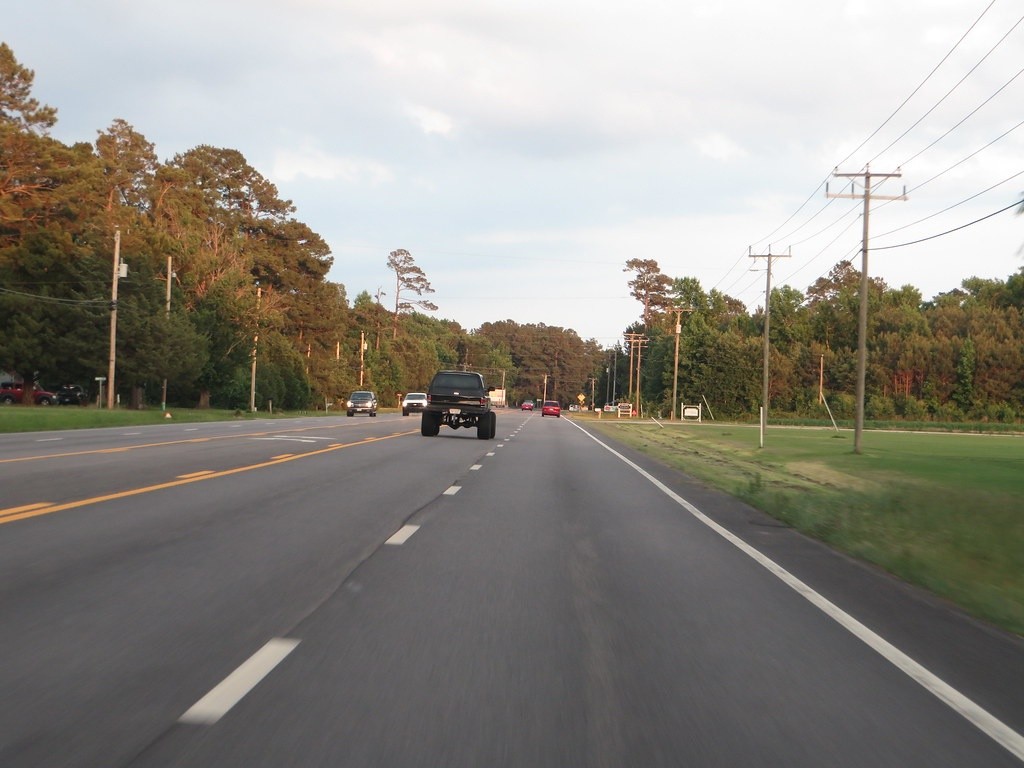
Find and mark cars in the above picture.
[521,401,534,411]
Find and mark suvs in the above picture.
[420,369,497,440]
[57,383,88,406]
[346,390,378,417]
[541,400,560,417]
[401,393,428,416]
[0,380,56,406]
